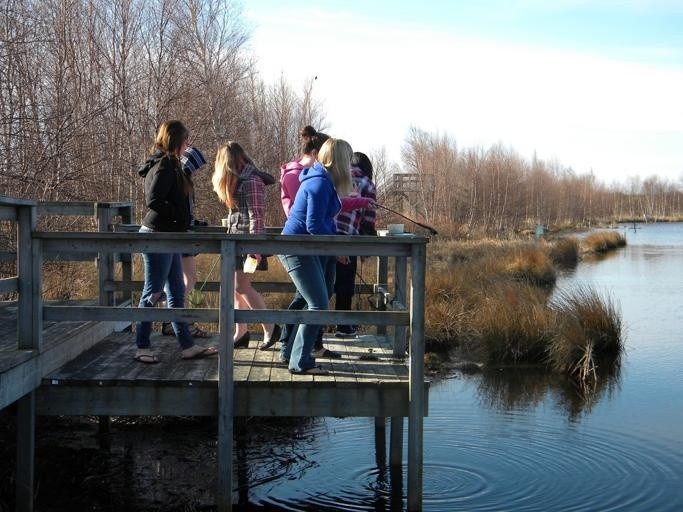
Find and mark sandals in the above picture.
[162,323,212,338]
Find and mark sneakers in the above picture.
[335,329,357,337]
[310,347,341,358]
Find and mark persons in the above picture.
[161,146,211,339]
[278,138,353,376]
[211,140,282,350]
[279,125,379,359]
[132,120,218,364]
[335,152,378,337]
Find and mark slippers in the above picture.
[133,355,159,363]
[181,349,218,360]
[302,365,329,375]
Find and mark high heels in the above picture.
[234,331,250,349]
[260,324,282,351]
